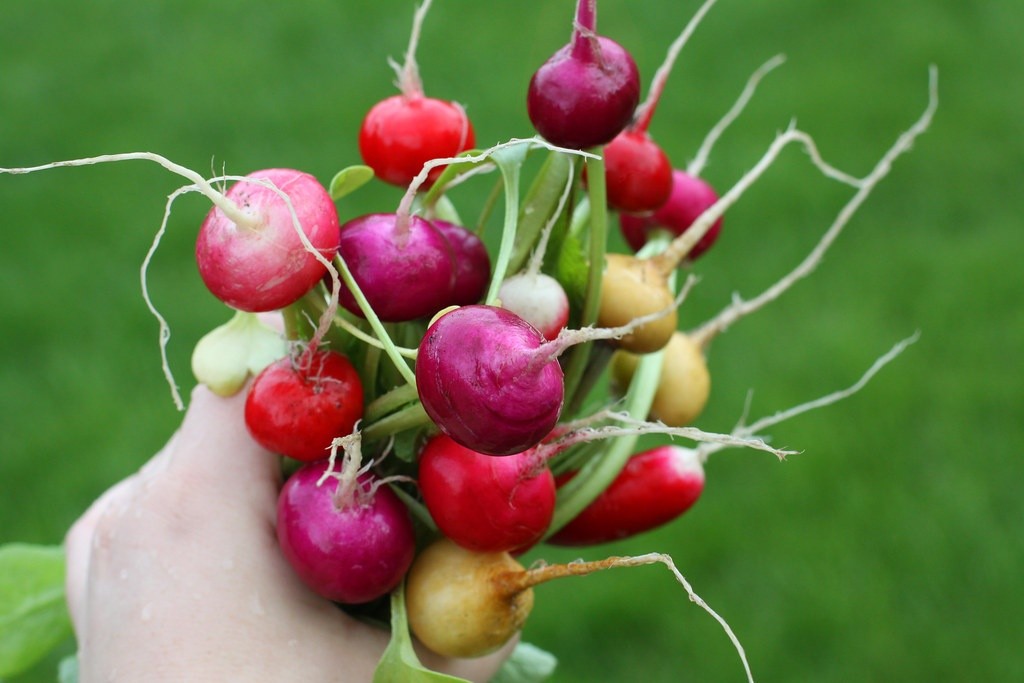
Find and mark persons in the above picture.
[66,310,521,683]
